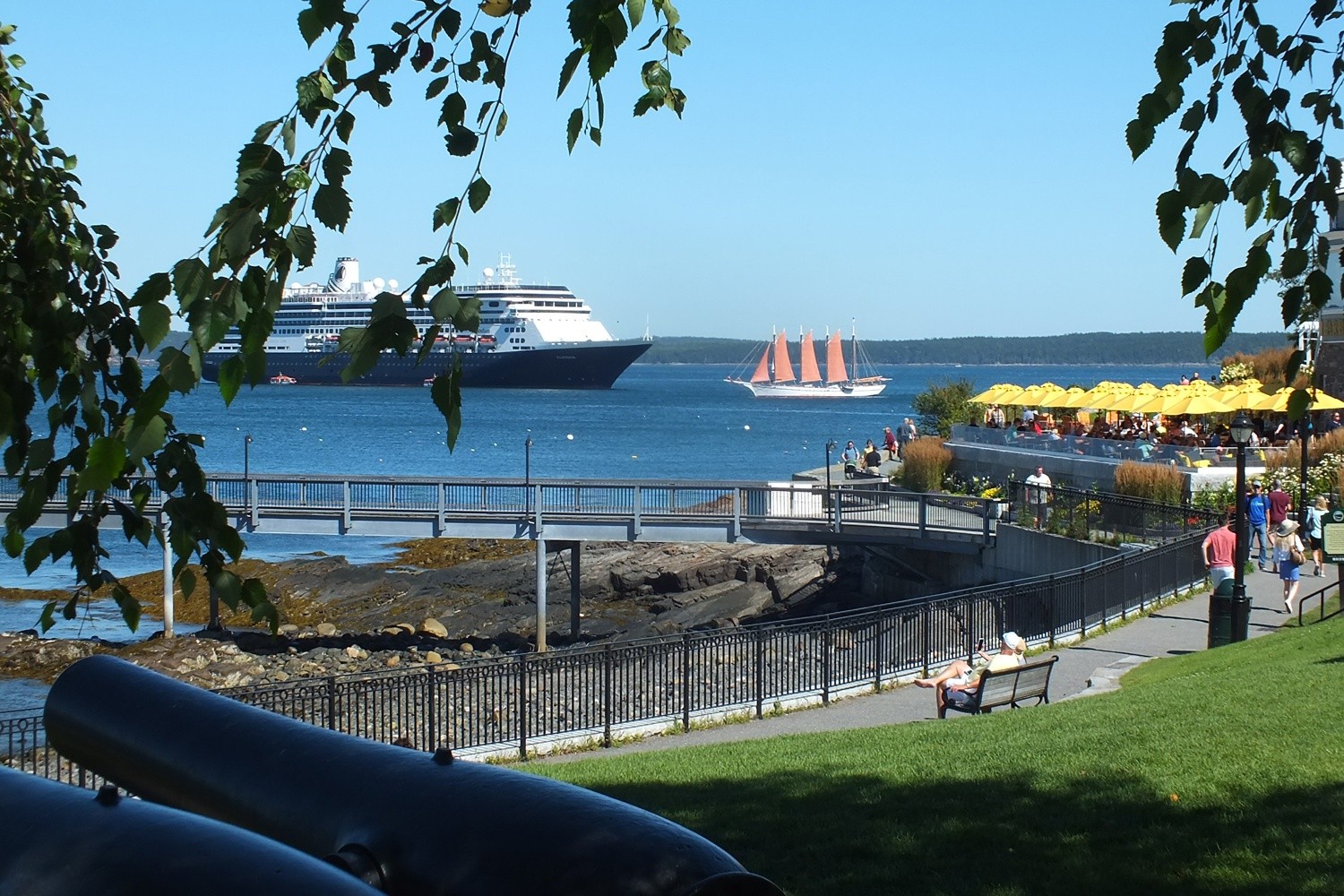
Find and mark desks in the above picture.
[1121,447,1137,461]
[1022,435,1037,449]
[1061,435,1082,454]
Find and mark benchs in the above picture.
[1192,453,1233,468]
[940,654,1060,718]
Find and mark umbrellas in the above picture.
[967,378,1344,416]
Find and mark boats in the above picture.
[478,337,495,346]
[423,373,437,388]
[325,333,341,342]
[453,337,476,347]
[270,371,297,385]
[411,336,450,348]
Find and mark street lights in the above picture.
[524,435,534,516]
[1228,410,1256,641]
[245,434,255,512]
[826,437,839,524]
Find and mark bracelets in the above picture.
[1267,524,1270,527]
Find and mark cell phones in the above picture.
[977,639,983,651]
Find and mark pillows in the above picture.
[1178,454,1192,467]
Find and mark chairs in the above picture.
[1049,439,1068,452]
[1138,449,1157,463]
[1000,435,1020,447]
[962,430,984,443]
[1100,445,1123,460]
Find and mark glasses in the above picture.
[1336,415,1340,417]
[1254,485,1260,489]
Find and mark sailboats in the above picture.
[723,316,893,399]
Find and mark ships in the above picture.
[200,252,654,392]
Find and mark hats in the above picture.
[1138,431,1147,439]
[1276,519,1300,536]
[1273,479,1281,485]
[1253,481,1261,486]
[1002,631,1020,650]
[1014,637,1026,659]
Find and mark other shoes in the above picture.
[1319,571,1326,578]
[1248,557,1252,561]
[1262,568,1269,571]
[1313,565,1320,576]
[1284,600,1292,614]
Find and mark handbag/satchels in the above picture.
[1290,550,1306,565]
[1306,508,1315,532]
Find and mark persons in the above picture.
[914,632,1027,718]
[1246,480,1270,572]
[842,441,860,462]
[1190,372,1199,385]
[1273,518,1306,614]
[897,417,916,462]
[862,438,881,476]
[1306,495,1330,578]
[1026,466,1052,530]
[1267,478,1292,547]
[882,427,894,450]
[1202,515,1237,595]
[964,404,1344,465]
[1180,375,1190,385]
